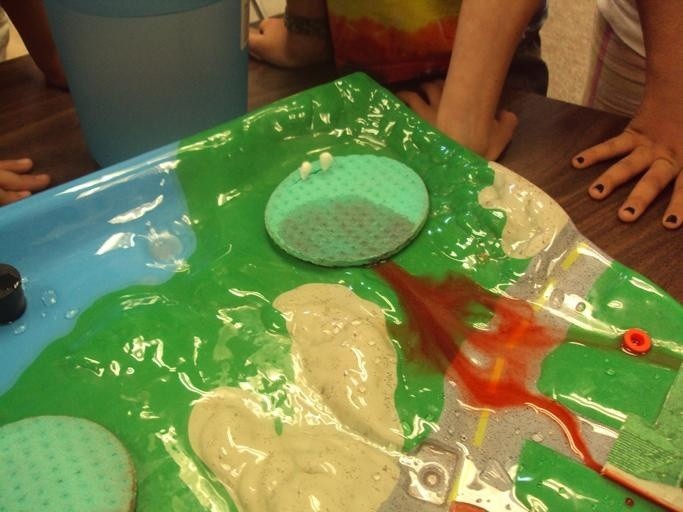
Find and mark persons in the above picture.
[570,1,683,230]
[247,0,550,162]
[0,0,70,94]
[0,157,49,204]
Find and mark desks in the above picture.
[1,79,683,510]
